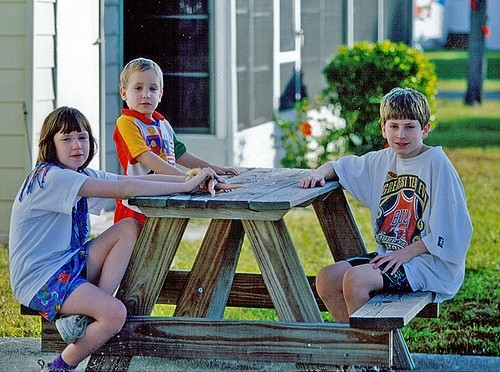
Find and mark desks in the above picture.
[20,166,421,372]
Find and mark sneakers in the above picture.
[36,359,51,372]
[55,314,92,344]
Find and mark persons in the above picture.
[112,57,241,225]
[296,87,473,372]
[8,107,218,372]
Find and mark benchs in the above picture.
[349,287,436,372]
